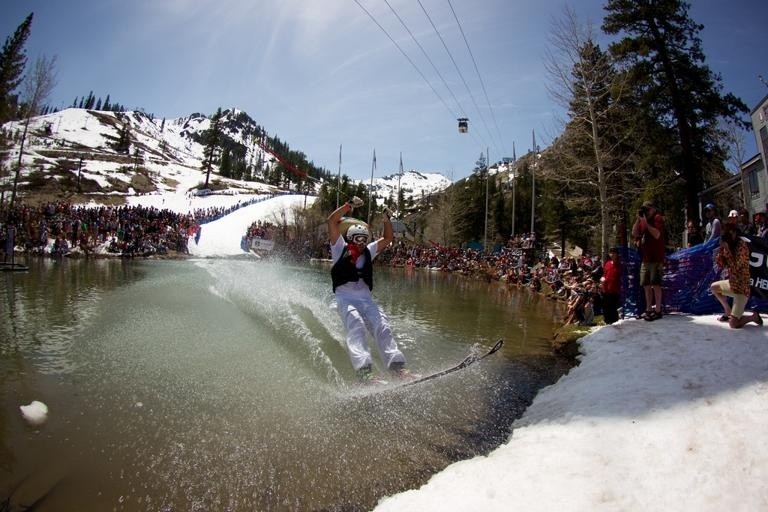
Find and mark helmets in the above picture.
[345,223,370,241]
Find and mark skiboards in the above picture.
[359,339,503,396]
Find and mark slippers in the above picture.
[753,311,764,326]
[717,315,729,322]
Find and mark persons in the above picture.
[0,196,225,258]
[323,195,420,388]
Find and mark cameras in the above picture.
[721,231,733,241]
[639,206,647,217]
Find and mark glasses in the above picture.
[351,234,368,245]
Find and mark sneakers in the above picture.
[358,372,391,389]
[390,368,424,384]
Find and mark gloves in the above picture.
[345,195,364,210]
[382,207,394,223]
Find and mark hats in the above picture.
[641,201,654,209]
[727,209,739,218]
[704,204,716,210]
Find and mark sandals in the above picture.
[636,312,664,322]
